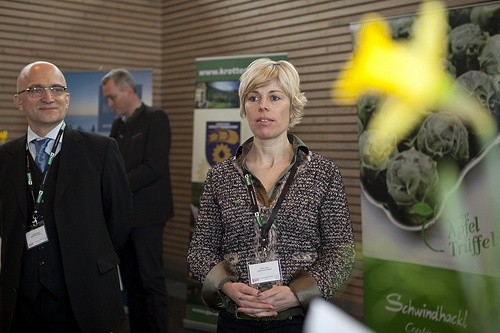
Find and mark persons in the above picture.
[101,68,176,333]
[0,61,133,333]
[186,58,357,333]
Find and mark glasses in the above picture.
[18,85,68,97]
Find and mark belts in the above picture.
[225,302,302,322]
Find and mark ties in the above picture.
[31,138,51,173]
